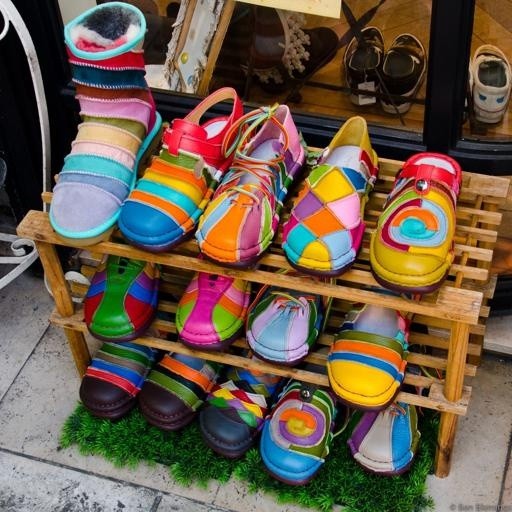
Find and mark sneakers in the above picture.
[343,27,385,108]
[257,27,339,94]
[380,32,427,115]
[463,44,512,124]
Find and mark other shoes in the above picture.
[194,102,304,269]
[280,116,378,277]
[117,87,251,253]
[245,270,341,366]
[175,253,259,352]
[199,350,297,458]
[370,152,461,293]
[347,363,430,476]
[79,341,158,418]
[83,226,161,342]
[138,338,232,431]
[325,285,423,411]
[259,346,346,486]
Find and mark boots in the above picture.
[48,1,163,248]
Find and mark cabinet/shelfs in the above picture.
[16,122,511,478]
